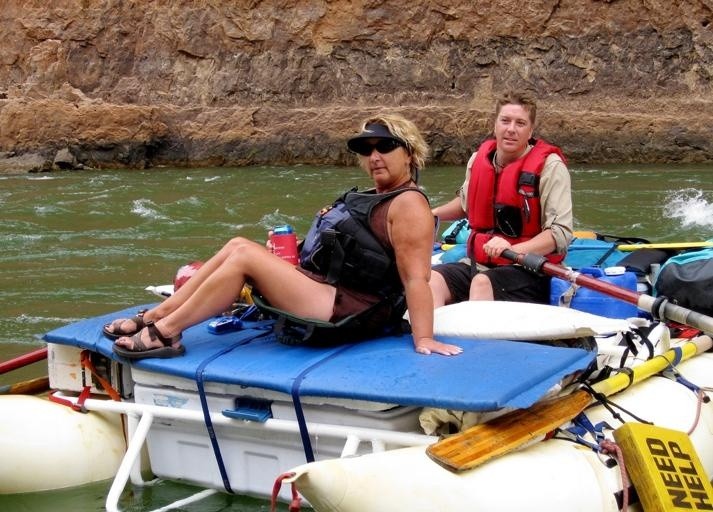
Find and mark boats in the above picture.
[0,229,713,512]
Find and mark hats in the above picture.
[347,122,404,148]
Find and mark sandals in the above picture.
[111,321,186,360]
[101,307,148,341]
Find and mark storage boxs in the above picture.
[46,340,133,399]
[133,382,450,508]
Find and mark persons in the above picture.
[100,115,464,359]
[429,89,574,311]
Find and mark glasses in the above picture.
[351,140,404,156]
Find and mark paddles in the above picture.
[441,240,713,250]
[426,335,713,475]
